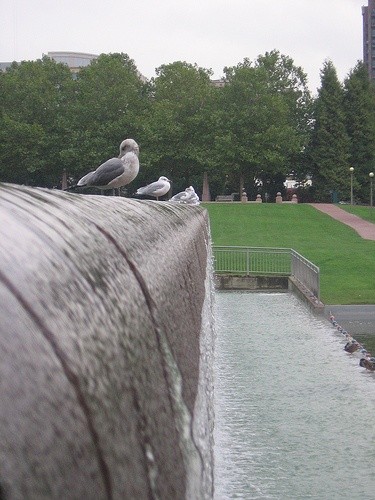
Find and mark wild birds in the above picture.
[359,358,375,371]
[137,176,172,201]
[64,139,140,196]
[169,185,199,202]
[344,340,360,354]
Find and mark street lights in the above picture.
[349,167,354,205]
[369,172,374,206]
